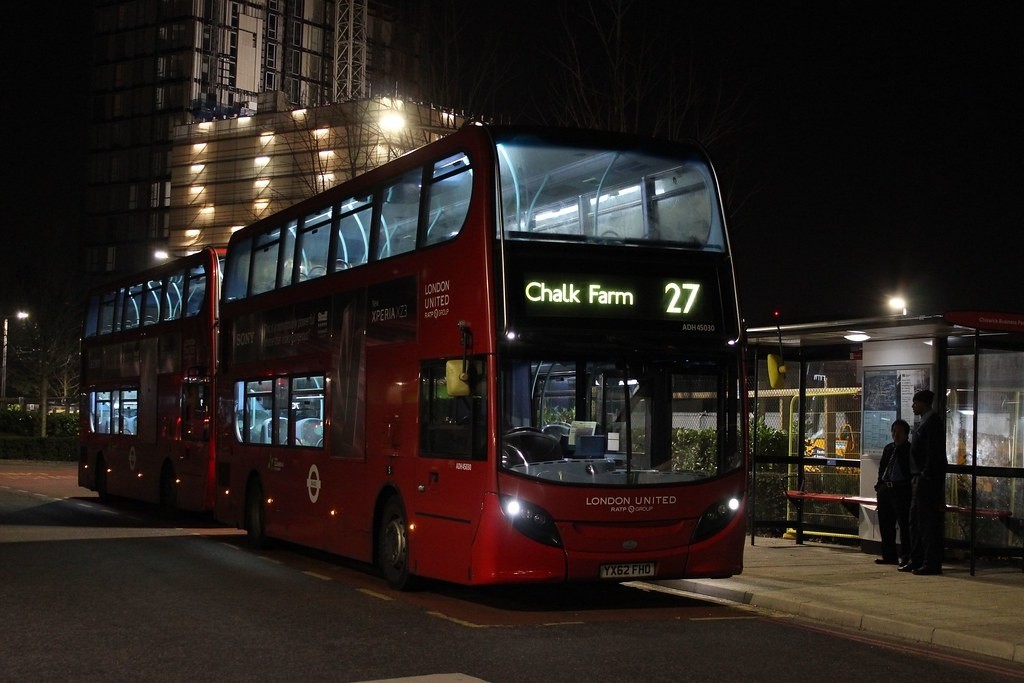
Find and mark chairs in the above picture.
[94,402,137,434]
[266,259,349,289]
[99,312,157,332]
[241,410,323,447]
[425,396,575,463]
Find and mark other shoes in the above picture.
[874,557,899,565]
[898,561,908,567]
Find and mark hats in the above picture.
[914,389,935,405]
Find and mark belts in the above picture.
[881,481,910,488]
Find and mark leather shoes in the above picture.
[896,564,916,572]
[911,566,933,575]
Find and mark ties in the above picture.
[881,447,897,480]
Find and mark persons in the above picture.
[898,388,947,574]
[445,361,490,424]
[871,419,916,566]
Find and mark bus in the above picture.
[78,244,228,521]
[214,121,751,592]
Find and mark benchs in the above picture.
[782,475,1012,576]
